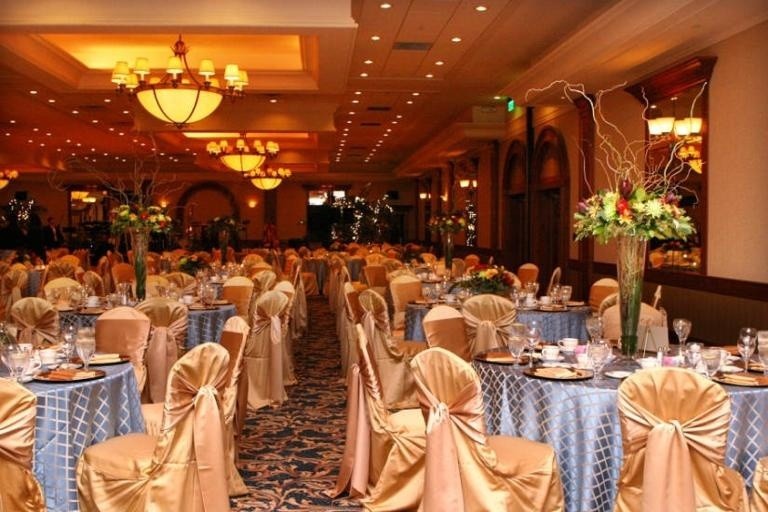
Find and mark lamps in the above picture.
[111,34,248,130]
[205,131,279,173]
[647,97,703,137]
[459,179,477,196]
[242,164,291,190]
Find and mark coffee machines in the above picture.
[17,343,32,356]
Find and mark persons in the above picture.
[43,216,65,243]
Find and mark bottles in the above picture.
[657,346,663,362]
[663,345,667,357]
[526,282,536,302]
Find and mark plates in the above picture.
[56,363,82,370]
[473,352,530,364]
[718,367,743,374]
[86,305,101,307]
[444,300,458,304]
[566,301,584,306]
[605,370,632,379]
[70,355,130,363]
[523,366,595,381]
[188,304,219,311]
[409,299,437,304]
[539,356,565,361]
[712,373,768,387]
[534,305,570,312]
[22,369,42,376]
[35,369,106,382]
[608,356,616,362]
[202,298,234,305]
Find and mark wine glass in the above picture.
[61,341,73,364]
[674,319,693,352]
[587,342,608,383]
[0,344,15,378]
[517,292,524,306]
[701,351,721,380]
[79,327,94,338]
[75,339,95,370]
[206,286,216,309]
[509,323,525,369]
[433,264,438,275]
[524,319,541,368]
[686,342,703,371]
[404,262,410,274]
[739,328,757,357]
[511,289,519,303]
[756,330,768,374]
[444,269,451,287]
[738,341,754,374]
[421,287,432,304]
[551,284,572,308]
[586,318,604,343]
[432,283,443,307]
[63,327,74,348]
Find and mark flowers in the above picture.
[109,203,172,297]
[524,77,706,333]
[426,210,468,269]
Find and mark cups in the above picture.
[87,296,98,304]
[210,276,217,281]
[184,296,192,304]
[541,345,559,358]
[661,351,681,369]
[718,350,730,364]
[557,337,578,349]
[446,293,456,301]
[540,296,553,304]
[10,354,31,387]
[526,298,537,307]
[26,359,40,371]
[40,350,57,365]
[527,300,536,306]
[608,348,613,357]
